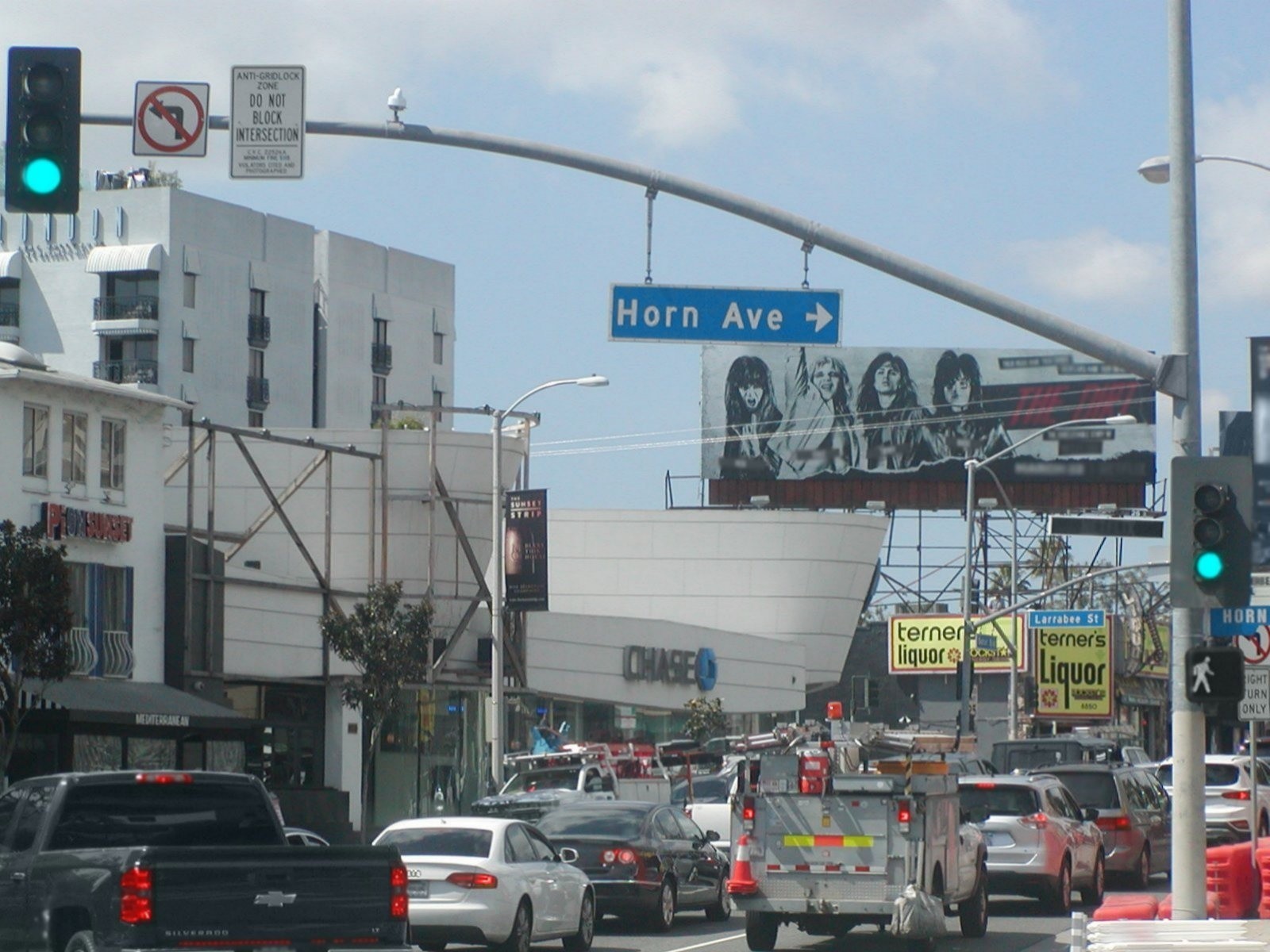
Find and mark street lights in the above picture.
[488,378,610,792]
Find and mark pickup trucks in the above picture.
[2,768,408,952]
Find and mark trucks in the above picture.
[730,702,992,952]
[471,739,671,826]
[989,737,1154,776]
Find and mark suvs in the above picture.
[884,753,1270,916]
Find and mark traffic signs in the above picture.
[230,65,307,180]
[132,81,209,158]
[607,283,844,349]
[1210,574,1270,721]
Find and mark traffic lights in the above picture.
[1192,481,1236,589]
[5,45,82,213]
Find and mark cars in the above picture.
[282,773,731,951]
